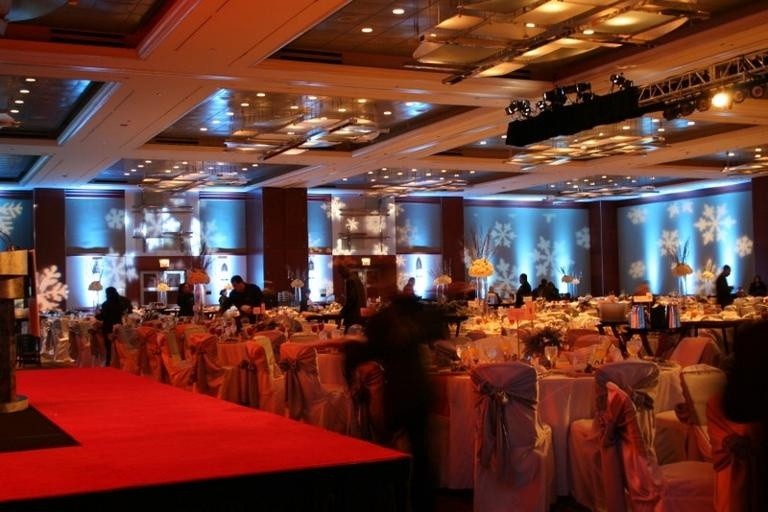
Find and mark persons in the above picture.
[715,265,733,306]
[176,283,195,317]
[486,286,500,305]
[516,273,531,308]
[218,289,228,306]
[748,273,766,296]
[531,279,570,300]
[298,288,310,313]
[633,280,648,295]
[338,263,450,511]
[333,263,361,326]
[402,276,415,296]
[94,286,132,368]
[215,275,264,321]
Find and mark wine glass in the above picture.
[48,291,768,375]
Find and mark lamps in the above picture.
[660,80,765,120]
[505,72,633,120]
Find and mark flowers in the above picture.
[562,275,581,284]
[432,275,452,285]
[155,282,170,292]
[186,271,210,285]
[87,281,103,290]
[700,270,715,280]
[290,278,305,289]
[468,259,495,278]
[671,263,693,276]
